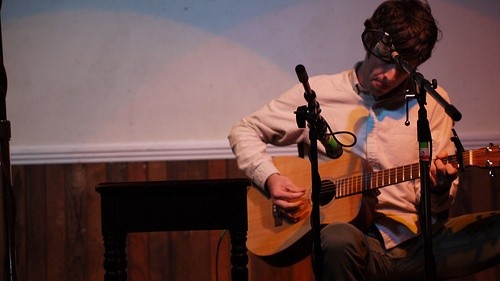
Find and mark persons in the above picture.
[228,0,500,281]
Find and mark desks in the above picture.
[95,178,251,281]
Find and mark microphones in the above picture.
[318,124,343,158]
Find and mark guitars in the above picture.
[245,142,500,266]
